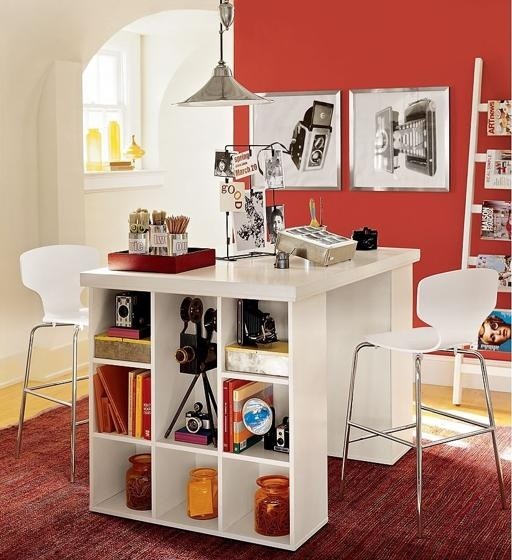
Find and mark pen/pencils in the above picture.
[164,214,190,234]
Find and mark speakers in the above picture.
[289,100,334,172]
[273,416,289,453]
[114,292,141,329]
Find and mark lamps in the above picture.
[172,0,272,108]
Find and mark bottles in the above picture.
[123,453,153,512]
[256,475,289,538]
[189,469,219,522]
[107,120,121,161]
[85,127,104,172]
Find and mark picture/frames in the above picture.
[347,87,449,194]
[249,89,342,192]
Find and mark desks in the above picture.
[78,243,423,554]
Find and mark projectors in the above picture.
[164,297,218,440]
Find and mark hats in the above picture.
[217,156,228,164]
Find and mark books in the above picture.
[484,149,512,189]
[487,99,512,136]
[475,253,512,292]
[127,369,152,441]
[222,378,274,454]
[274,225,358,267]
[479,200,512,240]
[476,310,512,352]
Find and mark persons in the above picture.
[269,209,284,245]
[214,157,228,177]
[479,315,512,345]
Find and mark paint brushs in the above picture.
[128,208,151,233]
[152,210,166,225]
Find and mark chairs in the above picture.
[19,248,109,483]
[341,268,508,538]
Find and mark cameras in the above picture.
[184,402,211,435]
[351,227,378,250]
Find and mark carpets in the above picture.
[0,397,512,560]
